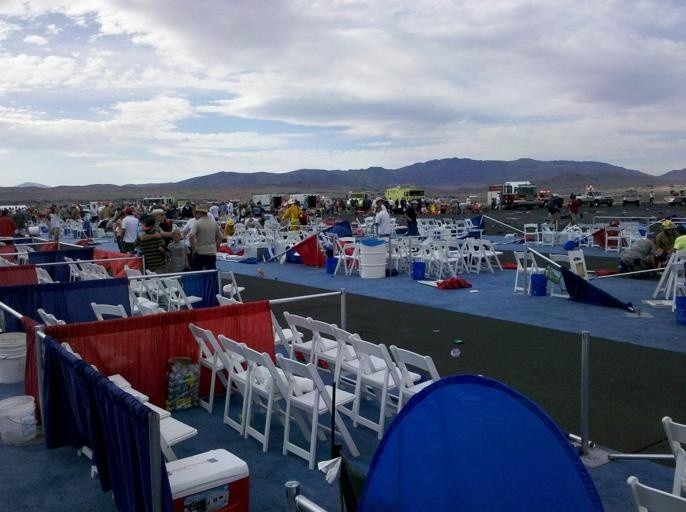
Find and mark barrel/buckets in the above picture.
[356,235,387,281]
[676,296,685,324]
[98,228,106,239]
[28,226,41,236]
[327,257,336,274]
[0,332,27,385]
[0,395,39,447]
[413,261,426,280]
[530,274,546,296]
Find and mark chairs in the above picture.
[626,415,686,512]
[512,221,642,299]
[653,248,686,312]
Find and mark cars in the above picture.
[621,189,643,207]
[664,194,686,206]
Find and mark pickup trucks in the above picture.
[572,190,614,208]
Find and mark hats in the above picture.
[195,204,208,213]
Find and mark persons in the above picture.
[620,214,686,270]
[364,194,441,214]
[570,199,582,225]
[334,198,346,210]
[451,196,459,214]
[90,198,223,270]
[401,200,419,236]
[374,197,391,236]
[466,195,479,213]
[209,199,265,226]
[649,191,656,204]
[278,199,308,230]
[1,203,85,241]
